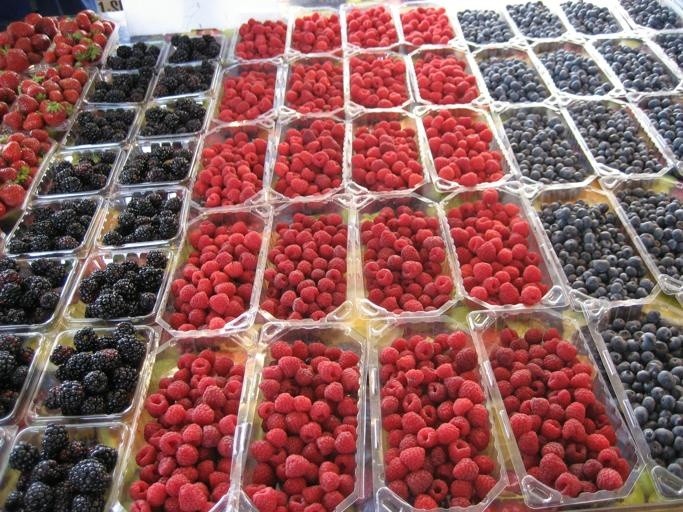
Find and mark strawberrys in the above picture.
[0,10,112,246]
